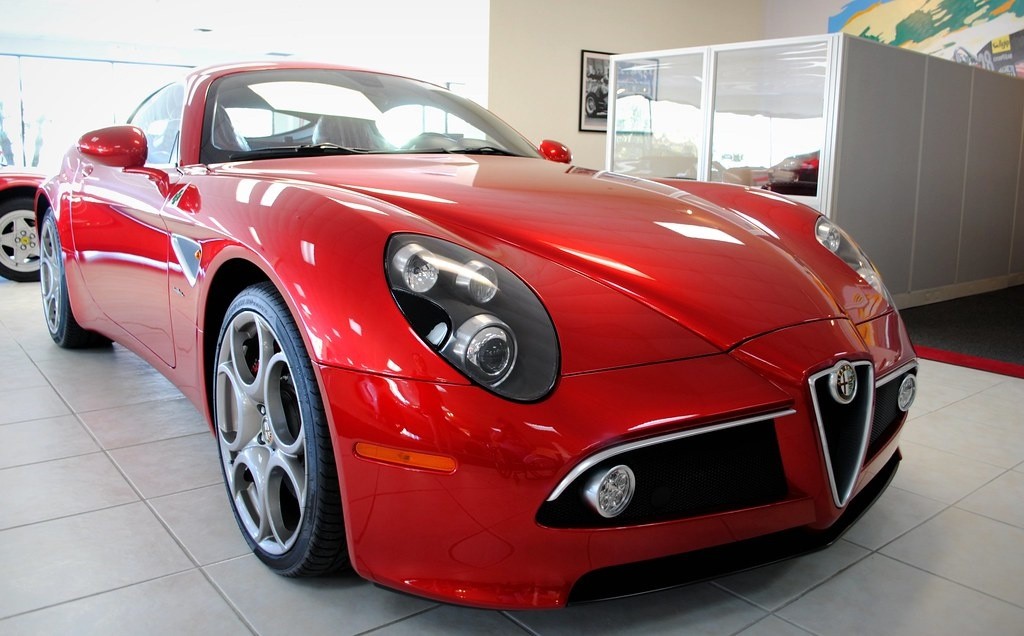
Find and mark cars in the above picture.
[36,60,920,612]
[0,145,60,282]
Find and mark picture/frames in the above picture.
[578,50,659,137]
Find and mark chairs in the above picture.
[162,100,251,165]
[312,115,396,153]
